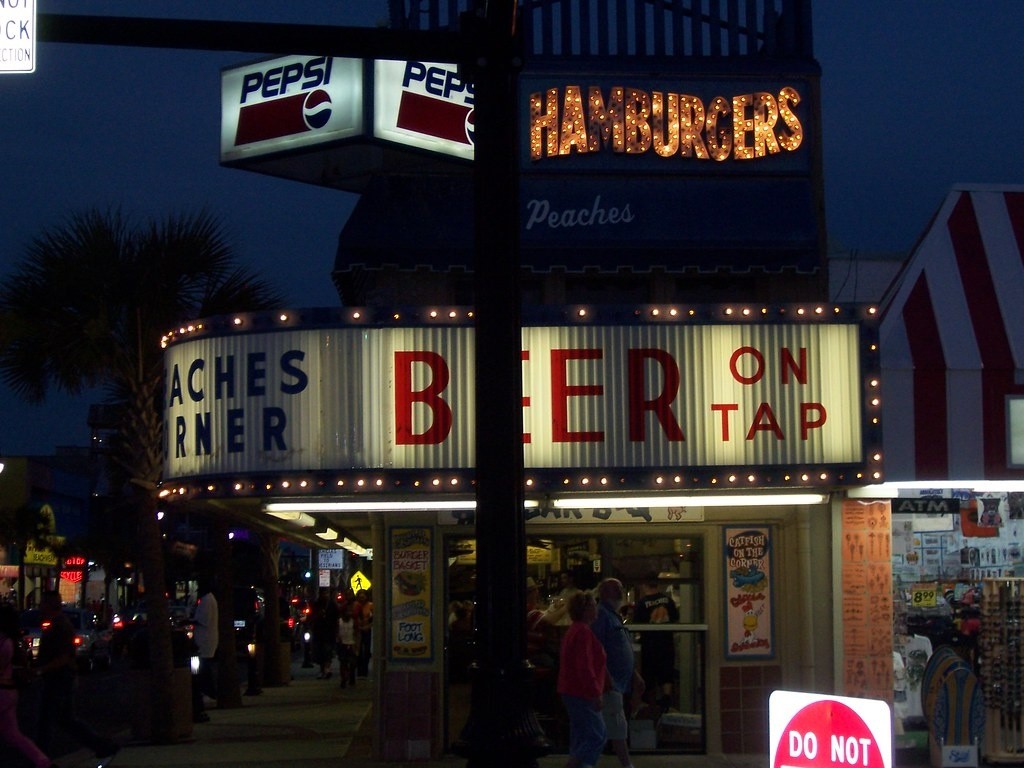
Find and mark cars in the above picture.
[14,606,114,675]
[110,601,262,668]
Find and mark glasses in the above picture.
[979,597,1024,715]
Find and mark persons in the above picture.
[335,604,360,690]
[540,570,585,626]
[0,600,59,768]
[557,590,610,768]
[168,615,210,723]
[589,577,646,768]
[85,593,114,627]
[118,594,126,610]
[18,609,121,768]
[353,589,373,678]
[310,587,338,680]
[619,568,680,714]
[177,577,219,708]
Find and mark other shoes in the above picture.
[96,747,123,768]
[317,672,331,679]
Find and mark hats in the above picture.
[527,577,543,588]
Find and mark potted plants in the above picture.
[106,612,291,745]
